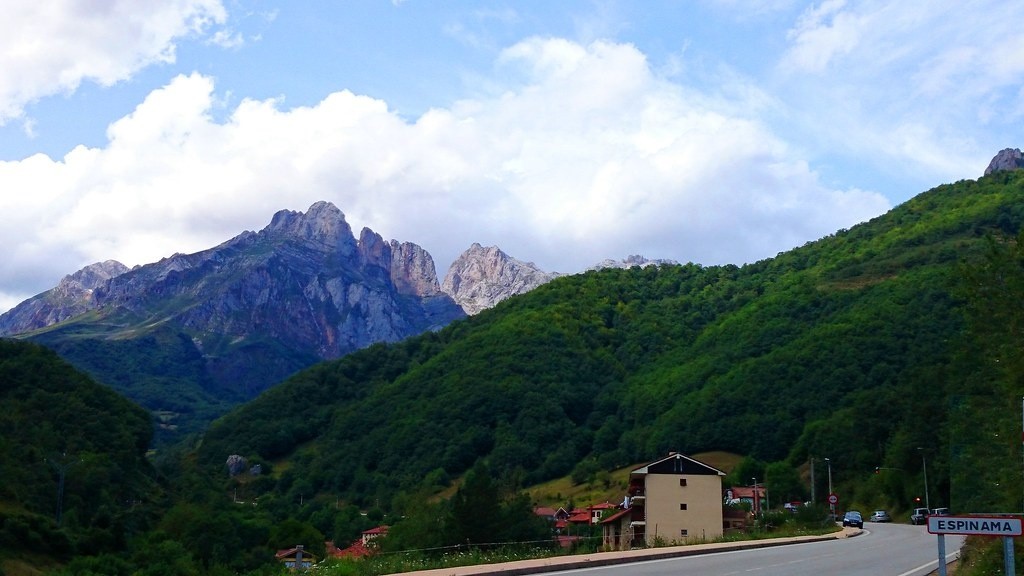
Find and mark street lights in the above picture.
[825,457,836,523]
[752,478,758,518]
[918,448,929,509]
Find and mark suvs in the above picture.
[911,508,930,525]
[931,508,948,517]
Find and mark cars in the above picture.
[843,510,863,529]
[870,511,891,523]
[784,503,800,513]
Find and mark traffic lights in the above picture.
[875,466,879,474]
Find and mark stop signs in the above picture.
[828,493,838,504]
